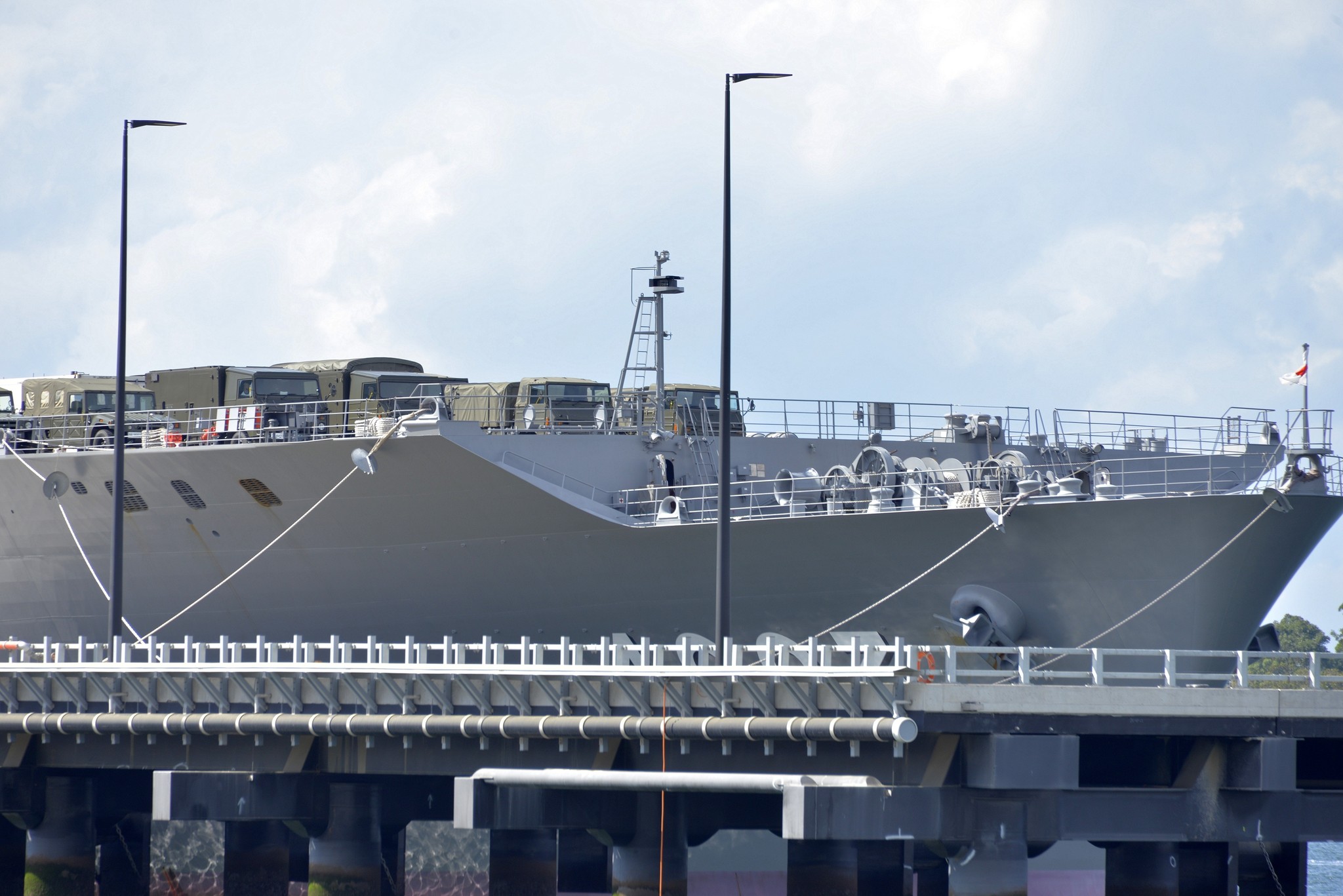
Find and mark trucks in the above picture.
[443,376,621,435]
[21,377,182,446]
[597,383,756,437]
[143,365,338,445]
[268,357,469,437]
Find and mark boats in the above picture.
[0,252,1340,825]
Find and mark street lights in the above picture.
[106,118,190,709]
[716,72,792,660]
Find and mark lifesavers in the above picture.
[917,651,935,684]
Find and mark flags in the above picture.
[1278,348,1309,387]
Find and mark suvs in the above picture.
[0,388,39,455]
